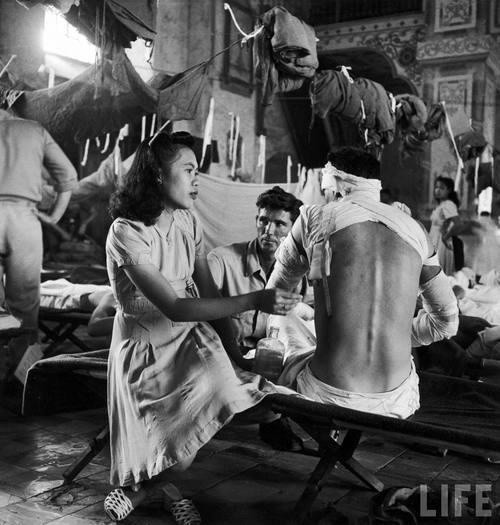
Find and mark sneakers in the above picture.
[257,418,304,453]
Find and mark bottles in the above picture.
[251,327,285,383]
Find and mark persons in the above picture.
[262,146,460,422]
[0,105,78,376]
[206,185,310,371]
[102,130,304,492]
[428,176,461,276]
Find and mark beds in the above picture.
[25,348,499,523]
[14,302,97,360]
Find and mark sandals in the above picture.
[158,482,204,525]
[103,477,139,522]
